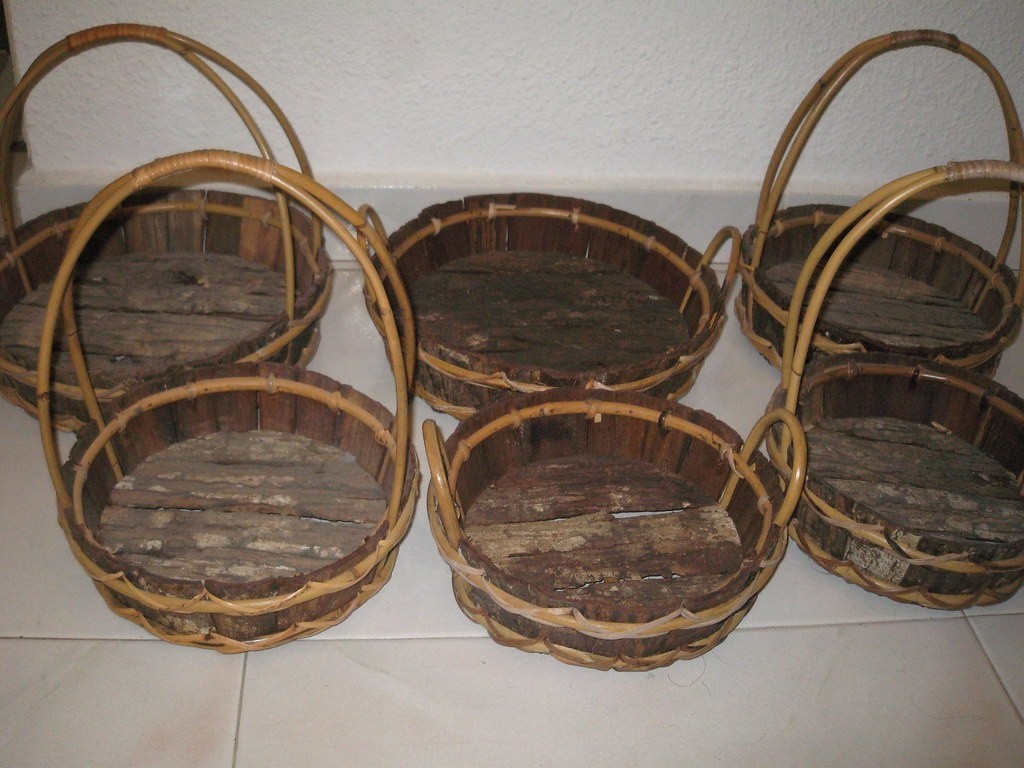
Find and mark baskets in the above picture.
[38,148,422,651]
[0,21,333,431]
[357,194,742,420]
[763,159,1024,608]
[735,30,1023,379]
[424,386,806,670]
